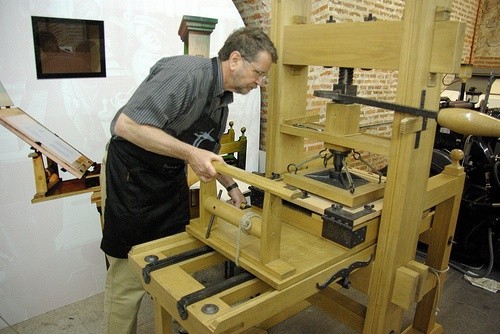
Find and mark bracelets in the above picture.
[227,183,238,192]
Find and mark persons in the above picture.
[100,28,278,334]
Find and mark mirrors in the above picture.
[31,16,106,80]
